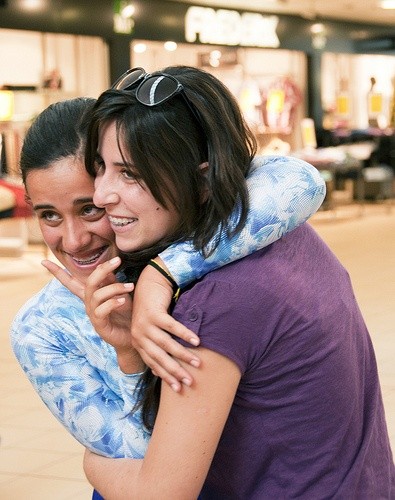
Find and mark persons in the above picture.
[251,75,382,126]
[83,66,395,500]
[9,98,327,500]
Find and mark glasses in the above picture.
[110,66,208,136]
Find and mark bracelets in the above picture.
[146,258,181,302]
